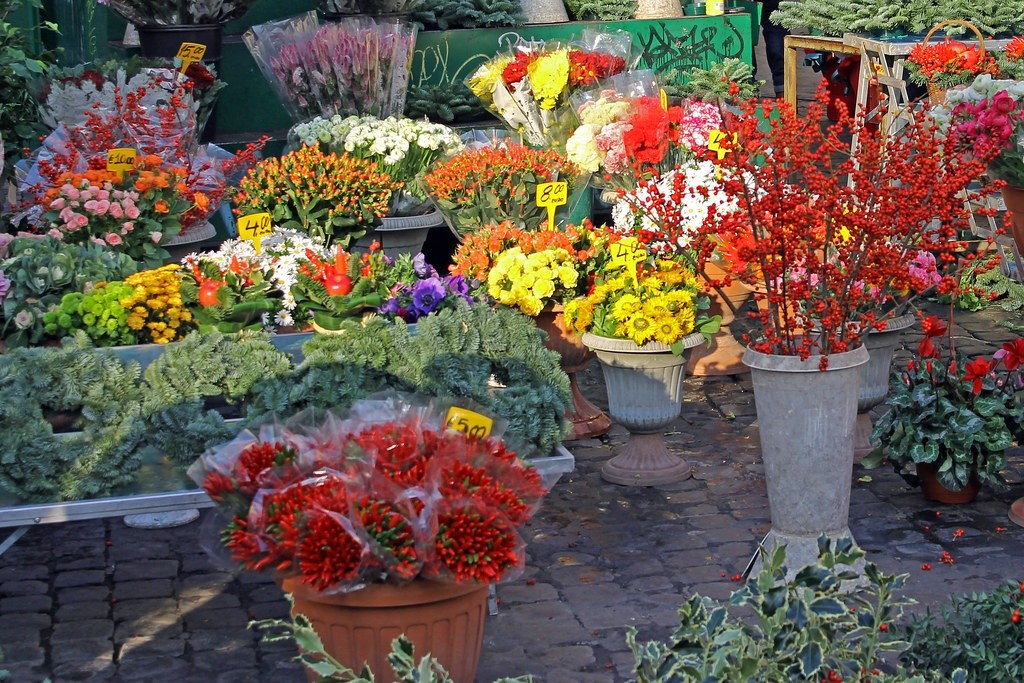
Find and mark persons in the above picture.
[746,0,791,100]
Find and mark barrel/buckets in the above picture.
[705,0,725,16]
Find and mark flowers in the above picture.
[0,17,1024,592]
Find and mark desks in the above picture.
[780,35,890,174]
[840,32,1021,191]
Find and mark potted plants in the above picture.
[868,358,1012,504]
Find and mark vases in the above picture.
[344,209,444,262]
[795,314,914,416]
[552,182,594,232]
[737,334,873,594]
[279,577,488,683]
[161,220,219,266]
[530,296,611,441]
[133,22,222,143]
[1000,187,1024,258]
[581,332,708,487]
